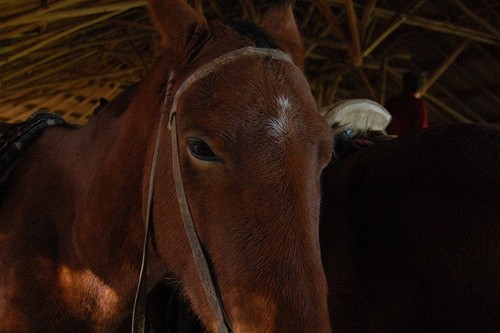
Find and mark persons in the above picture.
[386,72,429,134]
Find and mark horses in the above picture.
[0,1,393,333]
